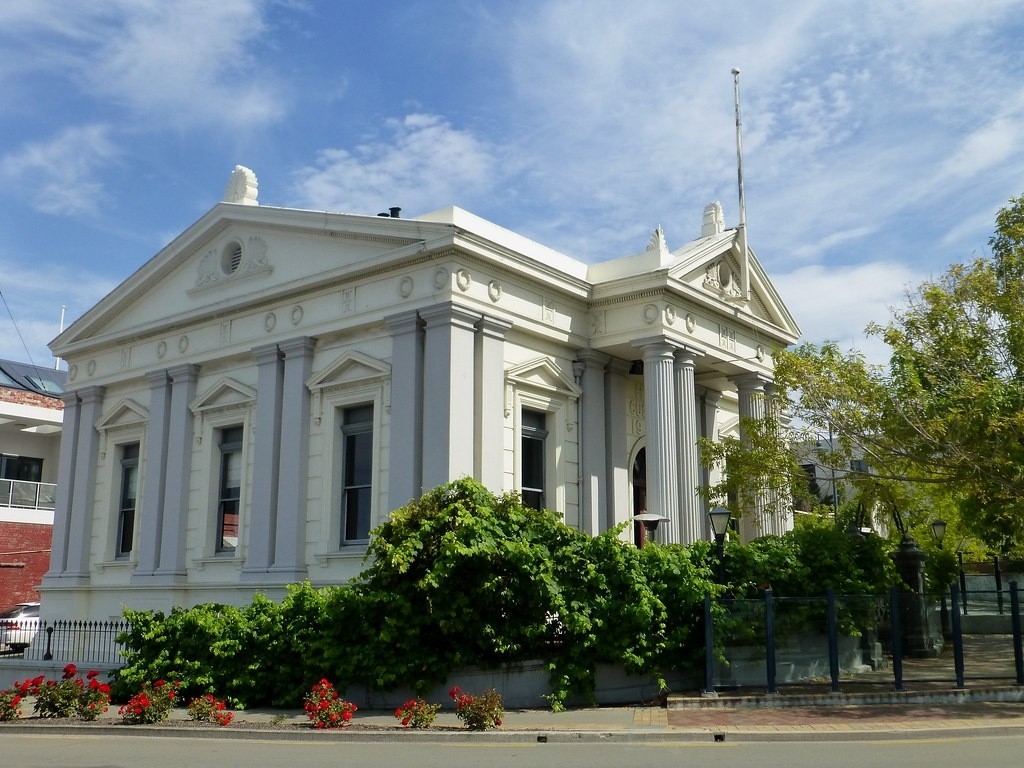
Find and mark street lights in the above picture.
[928,519,954,640]
[707,504,732,586]
[632,513,670,548]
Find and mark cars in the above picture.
[0,601,41,652]
